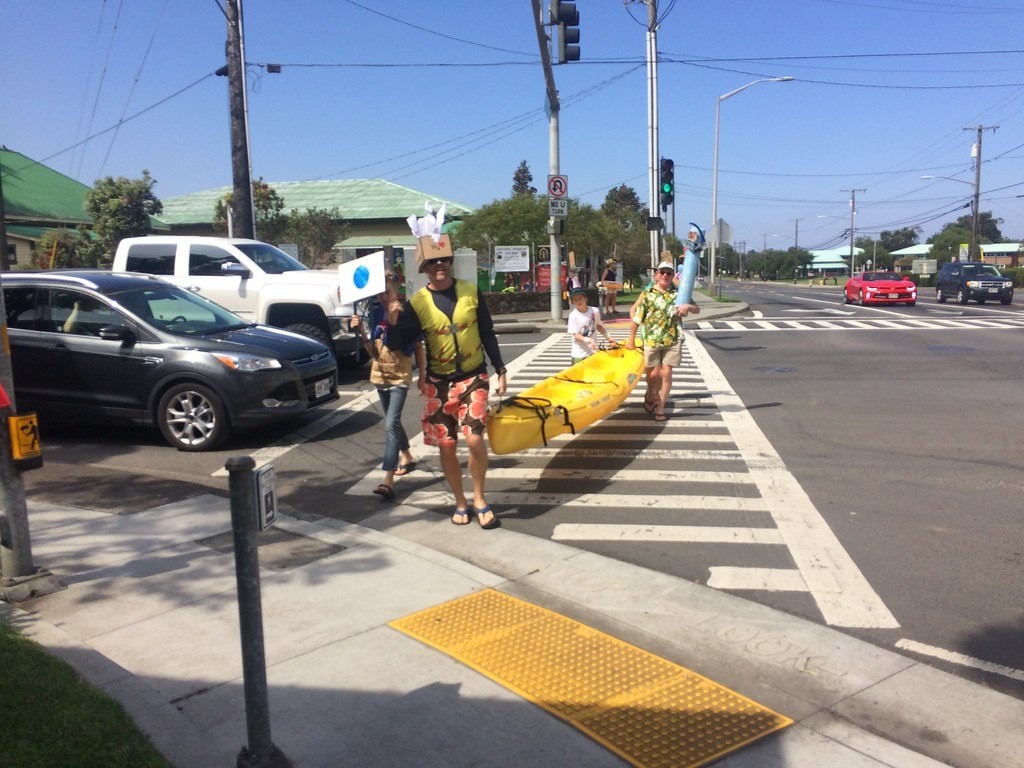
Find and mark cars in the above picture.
[807,271,815,278]
[844,270,917,307]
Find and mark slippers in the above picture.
[644,392,666,421]
[372,484,394,497]
[452,506,471,525]
[473,505,498,529]
[394,461,417,475]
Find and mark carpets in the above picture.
[386,588,797,768]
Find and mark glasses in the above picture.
[429,258,448,264]
[660,270,672,276]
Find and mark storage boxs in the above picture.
[415,233,454,274]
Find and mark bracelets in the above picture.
[362,338,370,344]
[604,332,610,339]
[583,337,591,344]
[496,367,507,375]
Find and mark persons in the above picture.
[567,287,618,366]
[626,262,700,422]
[385,232,507,530]
[565,269,581,292]
[503,272,533,292]
[601,259,620,316]
[351,268,428,497]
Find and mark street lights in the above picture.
[709,74,794,291]
[920,176,980,263]
[817,215,854,278]
[734,218,806,285]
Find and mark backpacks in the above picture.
[566,275,575,291]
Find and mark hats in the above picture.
[605,258,616,266]
[568,265,581,274]
[570,287,586,296]
[406,201,454,274]
[658,262,674,271]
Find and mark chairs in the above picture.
[116,291,154,319]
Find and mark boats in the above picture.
[486,337,647,456]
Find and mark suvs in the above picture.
[936,261,1013,306]
[0,269,340,451]
[112,236,372,371]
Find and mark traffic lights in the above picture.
[660,158,674,205]
[559,3,581,61]
